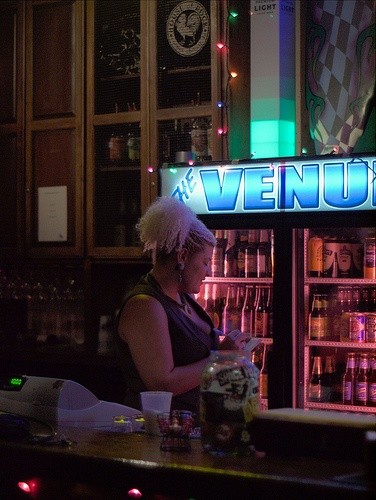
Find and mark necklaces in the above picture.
[174,291,193,315]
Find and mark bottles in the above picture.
[206,229,274,278]
[159,91,212,163]
[107,101,141,167]
[309,284,376,343]
[249,343,269,399]
[112,190,140,248]
[196,283,272,338]
[198,351,261,460]
[308,351,376,406]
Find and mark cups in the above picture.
[112,416,133,433]
[139,391,173,436]
[156,410,193,453]
[132,414,145,433]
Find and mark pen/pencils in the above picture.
[213,328,227,337]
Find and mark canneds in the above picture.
[339,312,376,344]
[322,237,376,279]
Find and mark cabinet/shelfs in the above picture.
[0,0,228,261]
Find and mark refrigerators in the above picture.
[157,151,376,420]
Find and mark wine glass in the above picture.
[0,264,85,306]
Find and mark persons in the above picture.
[114,196,263,426]
